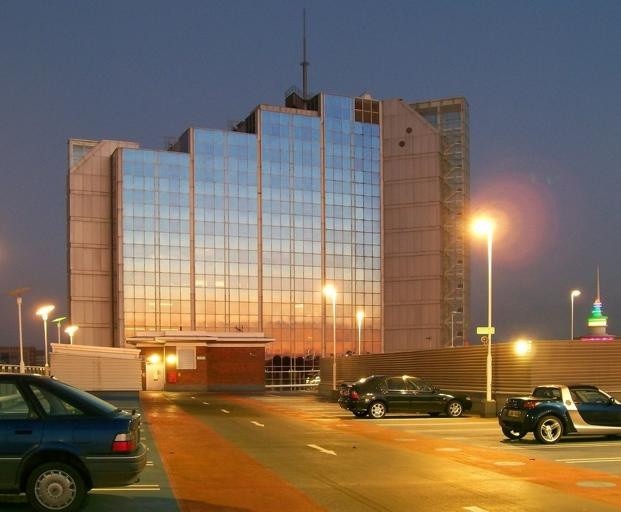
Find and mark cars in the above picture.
[0,372,147,512]
[338,374,472,419]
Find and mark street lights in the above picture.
[356,309,365,355]
[321,283,341,404]
[8,286,81,380]
[469,213,498,418]
[569,289,581,340]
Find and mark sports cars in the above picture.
[498,384,621,445]
